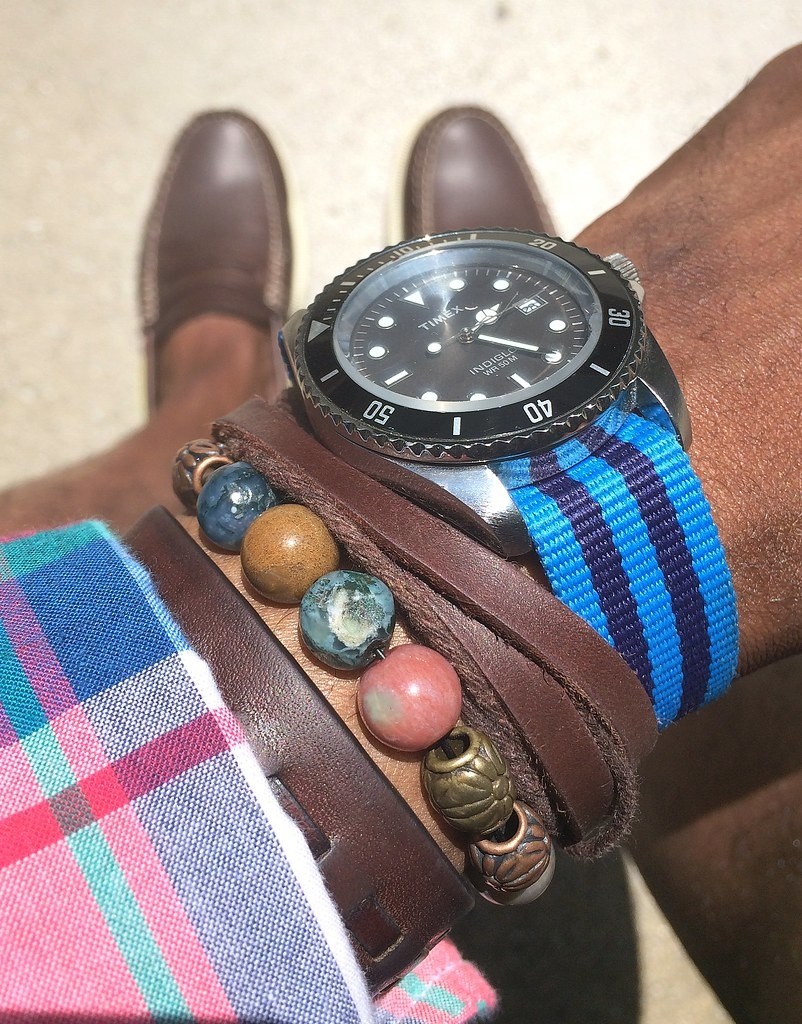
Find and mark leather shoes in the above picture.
[402,107,559,247]
[142,111,291,415]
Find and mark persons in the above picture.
[0,38,802,1024]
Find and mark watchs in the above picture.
[291,218,744,735]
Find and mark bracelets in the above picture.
[115,392,658,1006]
